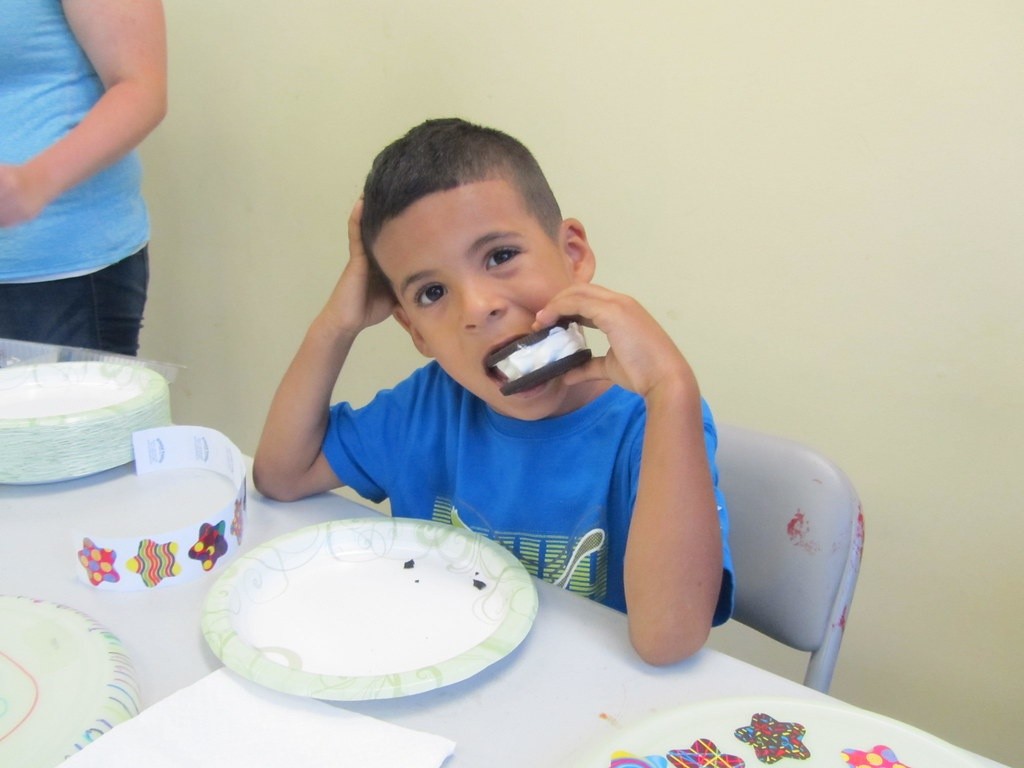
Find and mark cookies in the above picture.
[486,318,593,396]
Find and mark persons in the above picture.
[251,116,735,665]
[0,0,169,356]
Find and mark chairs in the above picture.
[712,424,864,693]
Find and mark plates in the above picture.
[0,362,172,485]
[200,516,538,701]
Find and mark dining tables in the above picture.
[0,455,1023,768]
